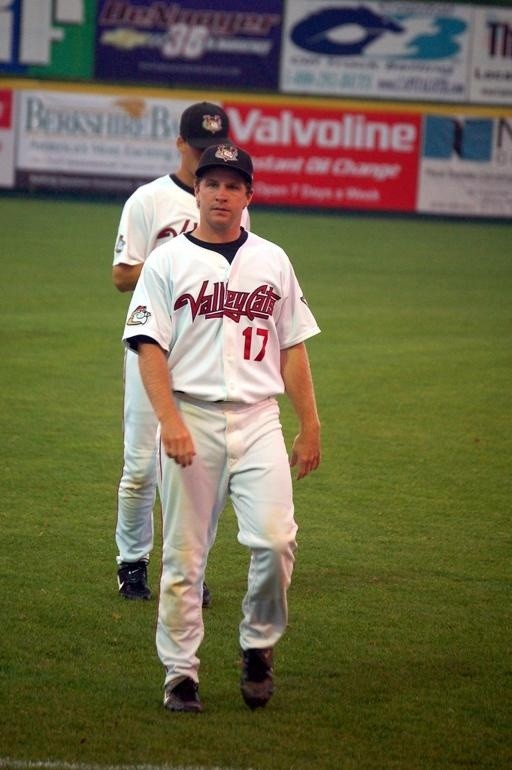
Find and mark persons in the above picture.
[114,101,251,605]
[123,144,320,712]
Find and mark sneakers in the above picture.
[114,558,153,599]
[198,573,216,608]
[239,636,276,707]
[160,670,204,713]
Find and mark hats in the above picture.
[177,100,237,150]
[192,140,254,183]
